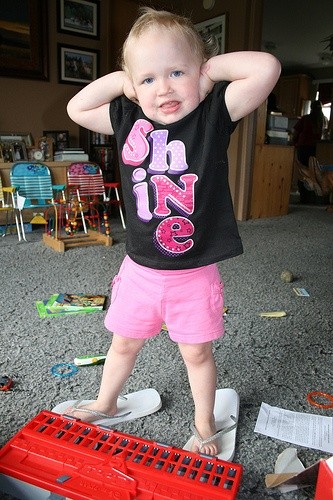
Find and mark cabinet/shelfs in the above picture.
[273,74,312,120]
[0,161,71,226]
[79,126,119,182]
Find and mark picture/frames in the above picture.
[0,0,52,83]
[56,42,101,86]
[56,0,101,41]
[193,12,227,55]
[0,132,35,162]
[43,130,69,149]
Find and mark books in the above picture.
[36,292,107,317]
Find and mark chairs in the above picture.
[0,162,126,243]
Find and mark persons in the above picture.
[55,7,281,457]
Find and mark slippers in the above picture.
[50,388,163,427]
[182,388,240,463]
